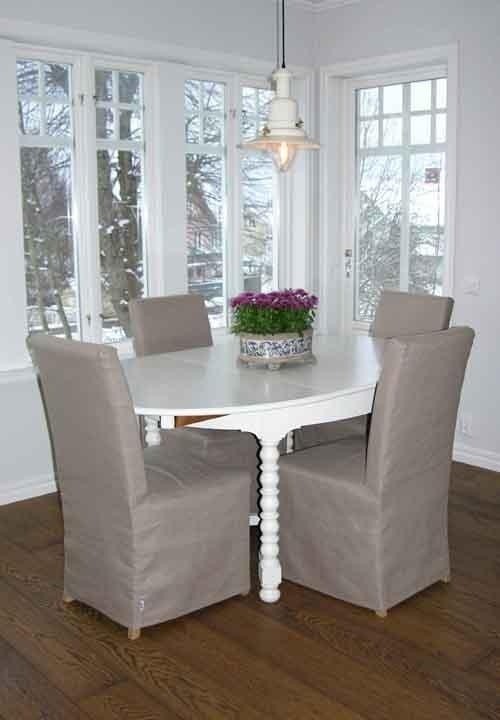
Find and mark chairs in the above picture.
[26,329,260,639]
[278,324,474,621]
[368,289,453,337]
[128,289,296,458]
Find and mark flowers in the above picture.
[228,287,319,330]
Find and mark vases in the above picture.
[236,329,316,371]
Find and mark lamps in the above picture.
[240,0,322,172]
